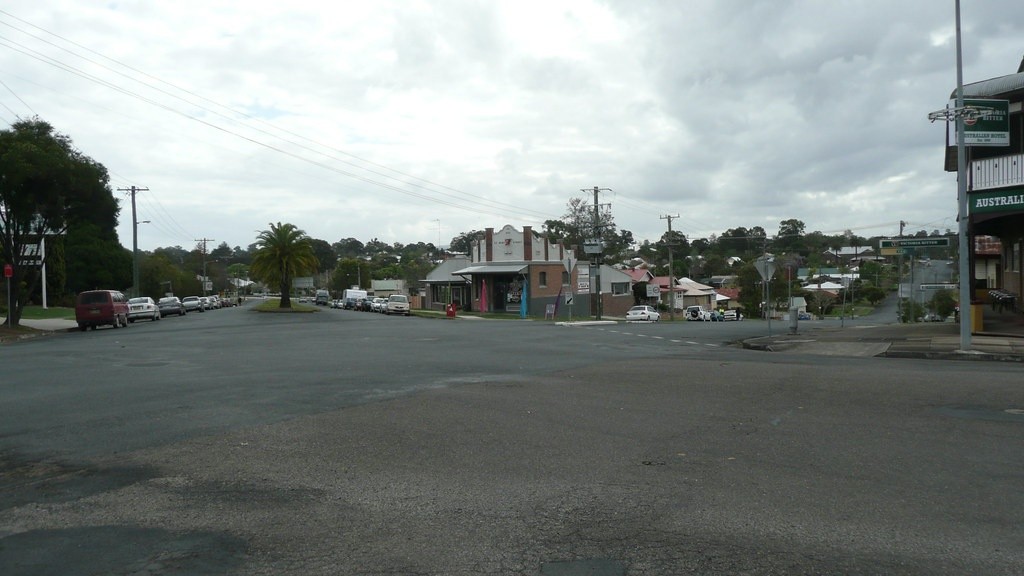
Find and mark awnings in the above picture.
[451,265,529,284]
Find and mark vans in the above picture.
[74,289,130,332]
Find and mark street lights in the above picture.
[897,220,907,323]
[203,259,219,297]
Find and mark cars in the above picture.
[200,294,233,310]
[625,304,661,324]
[158,297,187,316]
[125,296,161,323]
[710,311,724,322]
[298,286,413,316]
[181,295,205,312]
[724,309,743,321]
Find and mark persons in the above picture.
[719,306,725,317]
[735,307,740,321]
[238,298,241,306]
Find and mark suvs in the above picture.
[685,304,712,323]
